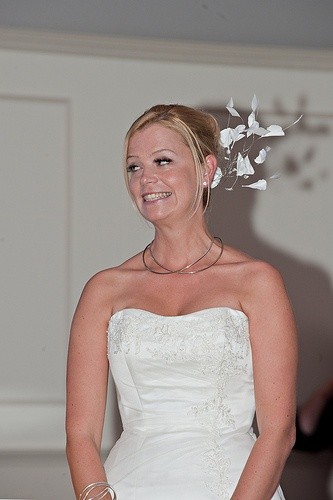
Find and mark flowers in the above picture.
[210,96,302,191]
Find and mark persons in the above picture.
[64,103,300,500]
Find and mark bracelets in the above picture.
[78,481,117,500]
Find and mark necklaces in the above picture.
[142,233,225,275]
